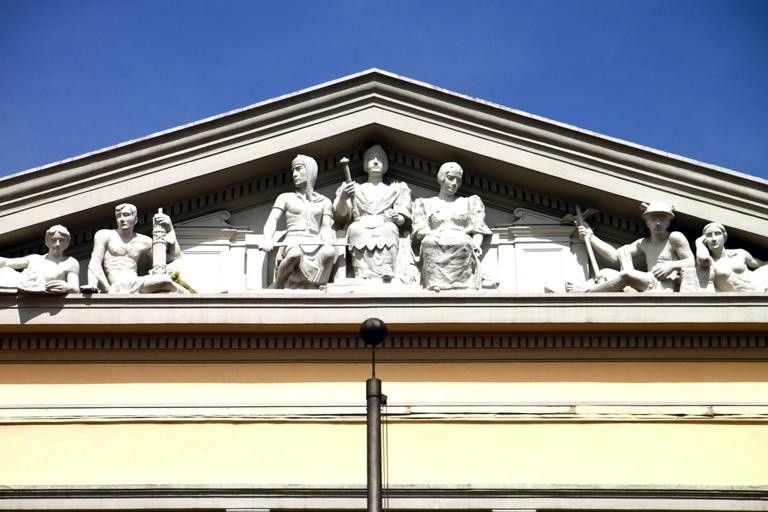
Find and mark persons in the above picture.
[407,159,494,291]
[253,153,339,293]
[79,200,229,295]
[330,142,412,281]
[692,221,767,295]
[542,198,695,294]
[0,221,83,297]
